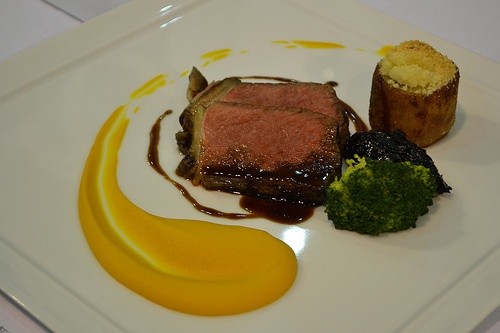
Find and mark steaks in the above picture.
[176,75,351,209]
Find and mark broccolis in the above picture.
[324,154,437,238]
